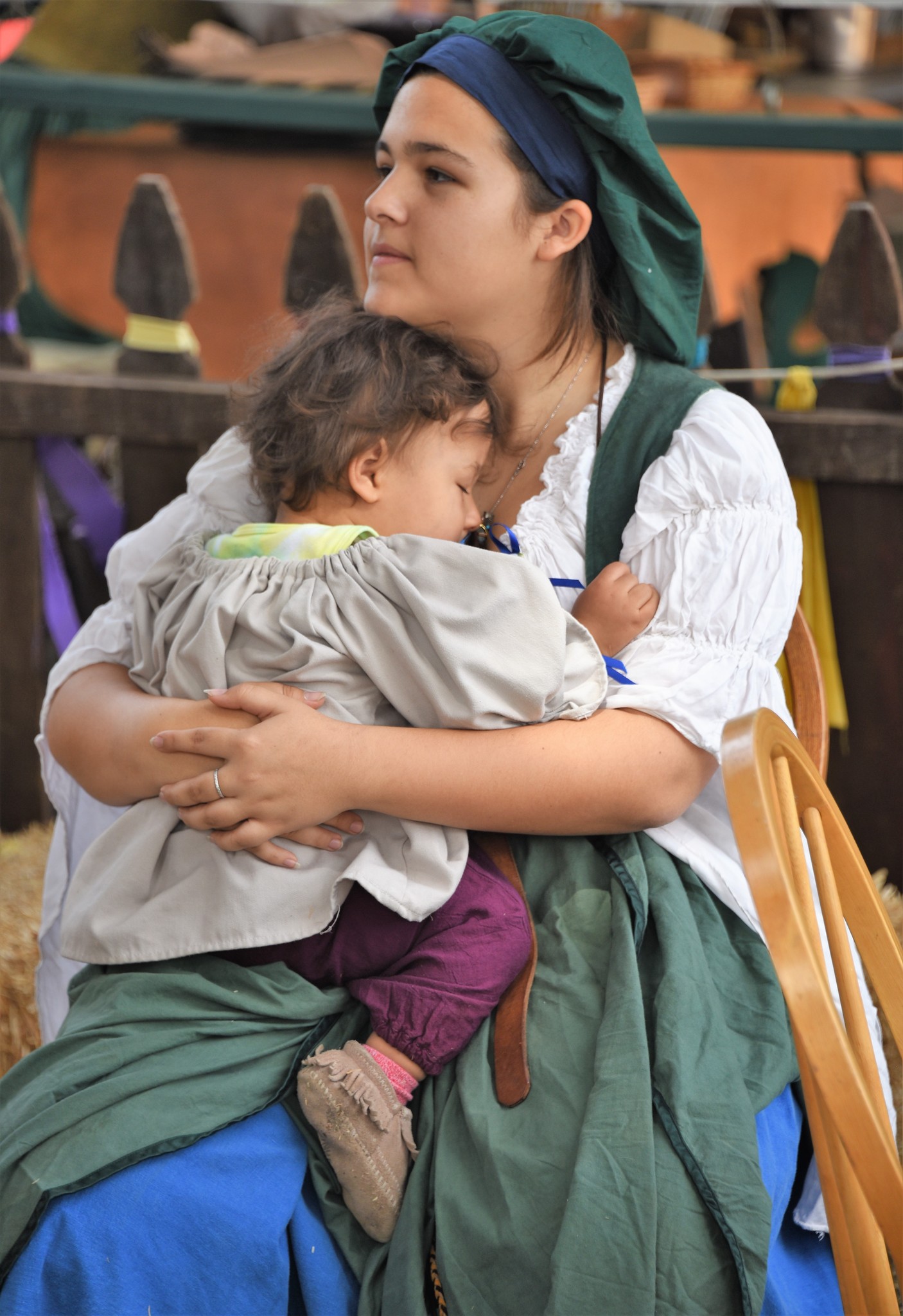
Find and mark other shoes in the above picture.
[296,1040,420,1246]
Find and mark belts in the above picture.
[486,848,532,1109]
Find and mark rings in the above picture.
[214,768,225,799]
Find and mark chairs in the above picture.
[723,706,903,1316]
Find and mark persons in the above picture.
[54,300,660,1247]
[0,9,900,1316]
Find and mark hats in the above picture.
[372,11,703,365]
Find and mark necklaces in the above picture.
[469,330,602,530]
